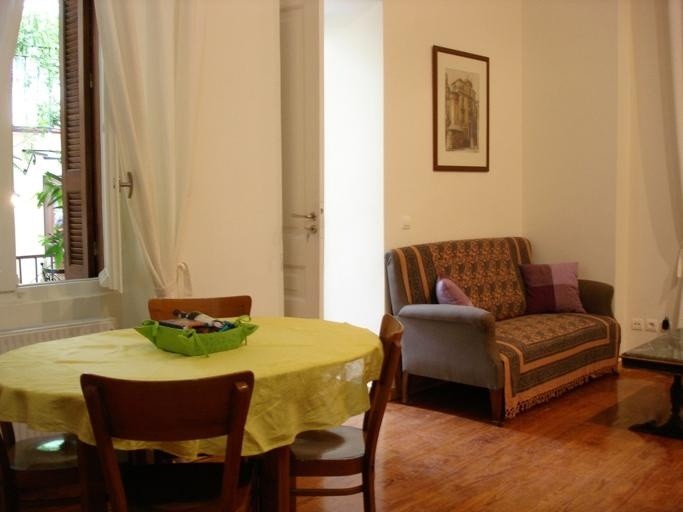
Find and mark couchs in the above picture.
[386,234,622,422]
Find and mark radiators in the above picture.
[0,319,114,442]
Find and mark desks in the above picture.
[0,313,384,510]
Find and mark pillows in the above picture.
[518,259,587,314]
[432,275,473,310]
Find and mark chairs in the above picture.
[0,420,94,511]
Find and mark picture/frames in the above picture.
[432,46,491,173]
